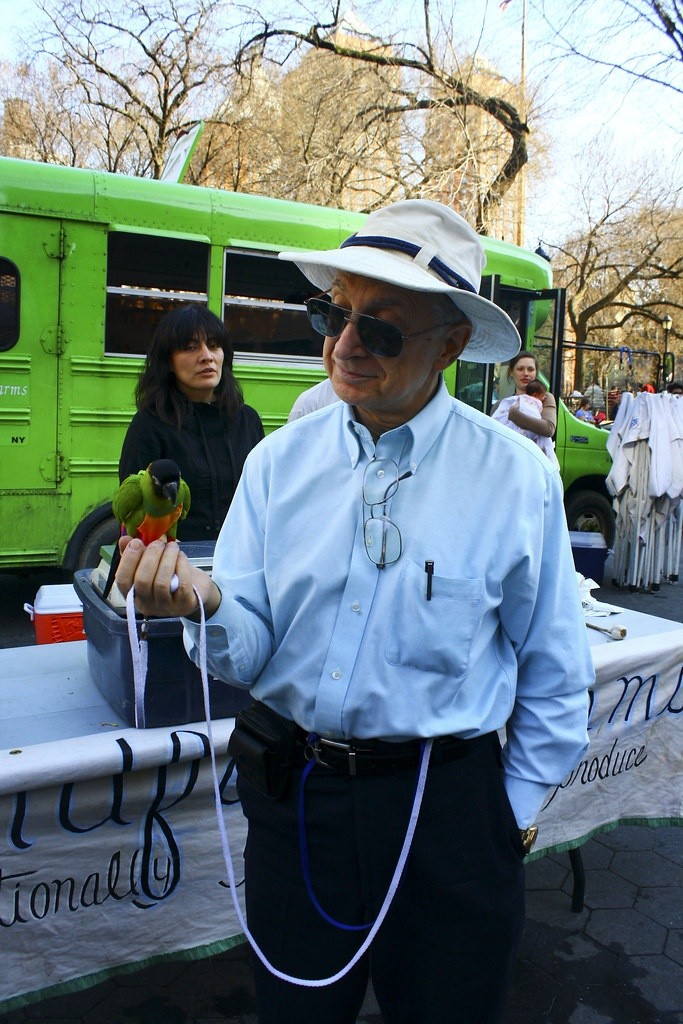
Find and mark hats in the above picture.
[278,200,522,364]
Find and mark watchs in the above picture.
[520,826,538,854]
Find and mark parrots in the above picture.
[100,458,192,601]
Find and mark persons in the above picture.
[119,302,266,543]
[570,379,683,424]
[114,199,595,1024]
[490,350,561,472]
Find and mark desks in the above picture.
[0,600,683,1024]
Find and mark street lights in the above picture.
[661,314,674,394]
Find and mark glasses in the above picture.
[361,458,413,570]
[304,289,458,360]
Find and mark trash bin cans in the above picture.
[23,584,88,645]
[569,530,608,595]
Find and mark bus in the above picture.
[1,154,625,595]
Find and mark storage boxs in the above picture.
[23,584,86,644]
[72,568,243,728]
[569,532,615,584]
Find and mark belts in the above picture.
[290,730,491,780]
